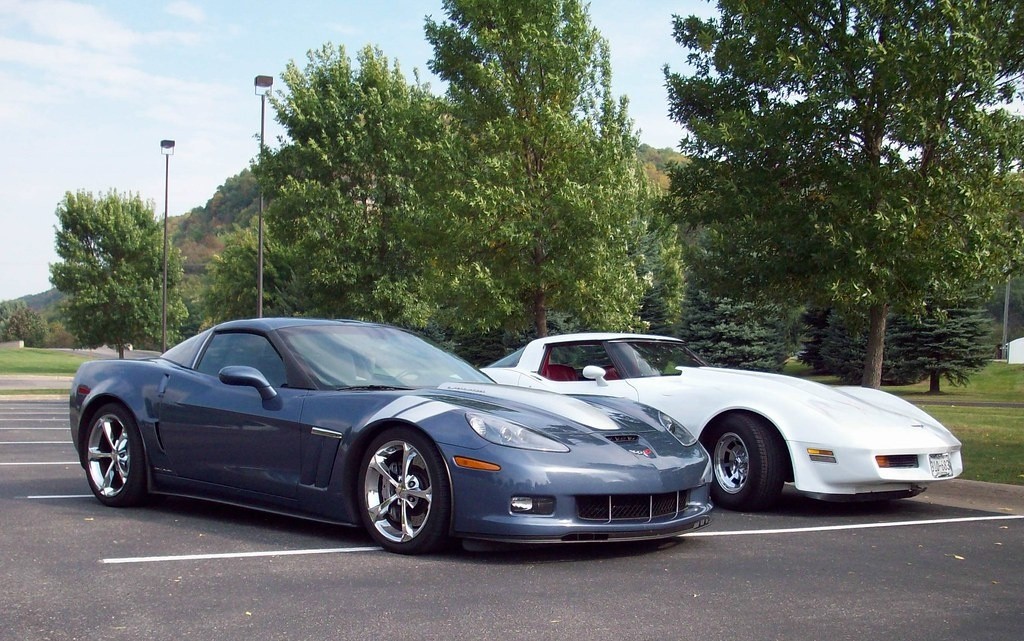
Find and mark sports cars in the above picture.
[69,318,715,557]
[449,333,962,514]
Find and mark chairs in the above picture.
[601,365,621,379]
[262,338,295,388]
[547,364,577,382]
[326,352,357,382]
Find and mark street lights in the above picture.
[161,140,175,353]
[255,76,273,319]
